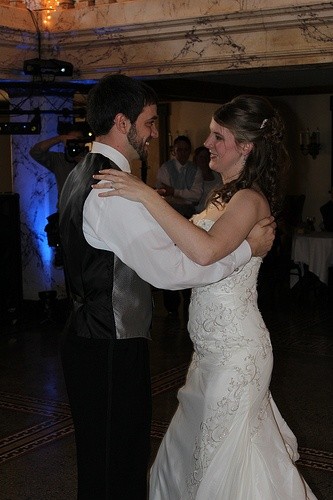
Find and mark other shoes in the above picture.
[165,311,181,336]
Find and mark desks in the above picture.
[291,232,333,301]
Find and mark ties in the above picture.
[178,168,187,175]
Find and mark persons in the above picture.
[56,73,276,500]
[91,96,317,500]
[31,116,333,500]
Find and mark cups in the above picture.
[156,183,165,199]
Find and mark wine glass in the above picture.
[305,216,316,232]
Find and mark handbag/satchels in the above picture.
[45,213,61,246]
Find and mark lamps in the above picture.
[300,127,321,160]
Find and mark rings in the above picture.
[110,181,114,189]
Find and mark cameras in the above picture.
[65,139,87,157]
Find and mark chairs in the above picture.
[284,194,306,230]
